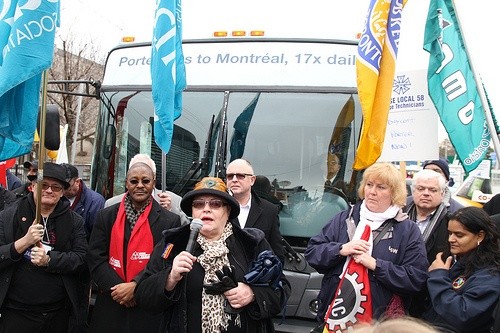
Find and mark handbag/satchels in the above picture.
[244,250,283,319]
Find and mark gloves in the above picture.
[203,264,244,315]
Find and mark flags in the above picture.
[424,0,500,173]
[204,92,261,175]
[319,225,373,333]
[0,0,61,162]
[351,0,407,171]
[150,0,186,154]
[322,96,355,203]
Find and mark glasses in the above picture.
[192,199,229,209]
[323,159,340,164]
[128,179,152,185]
[423,168,441,173]
[225,173,253,179]
[42,182,63,192]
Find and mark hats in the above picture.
[423,158,450,181]
[59,163,79,182]
[23,160,39,169]
[28,162,70,189]
[180,177,241,220]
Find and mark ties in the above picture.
[324,181,331,192]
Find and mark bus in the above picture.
[37,30,381,333]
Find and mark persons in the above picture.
[306,154,348,199]
[305,159,500,333]
[0,158,292,333]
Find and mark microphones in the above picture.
[184,218,204,253]
